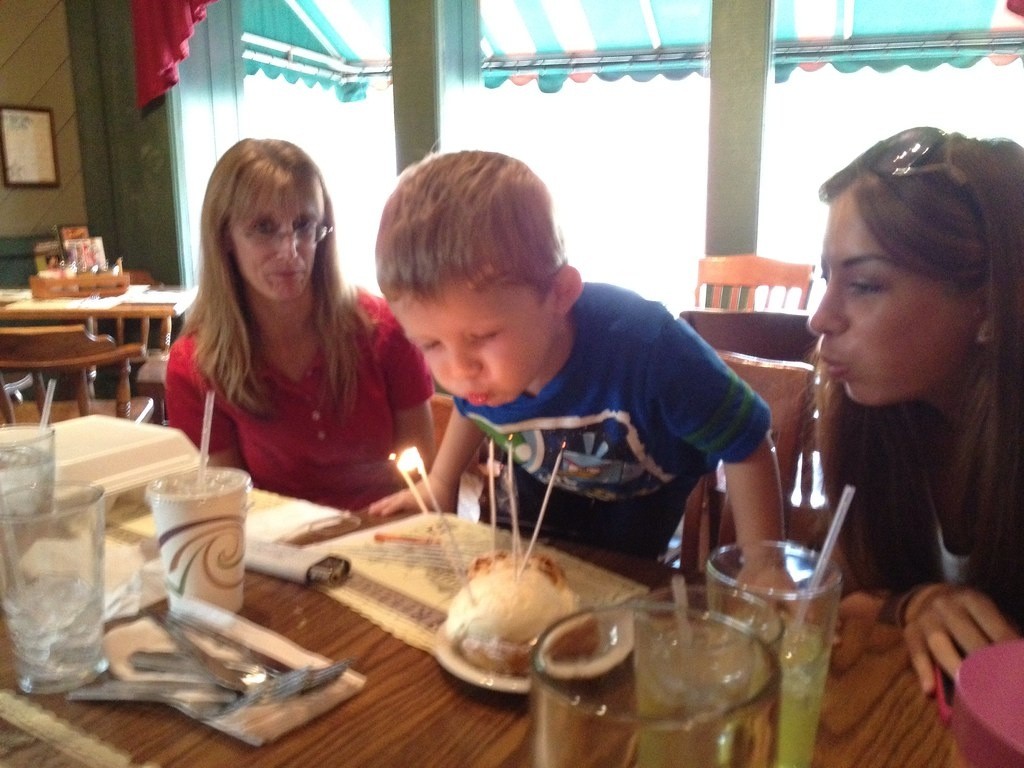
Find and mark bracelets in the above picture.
[877,589,916,627]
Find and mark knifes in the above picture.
[64,608,250,704]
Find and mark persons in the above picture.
[367,150,797,591]
[166,138,436,511]
[809,127,1024,697]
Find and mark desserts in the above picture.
[446,552,598,668]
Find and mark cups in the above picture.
[530,539,840,768]
[145,466,253,614]
[0,478,108,696]
[0,423,56,491]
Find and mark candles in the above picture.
[387,429,571,608]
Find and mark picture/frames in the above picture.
[0,102,61,189]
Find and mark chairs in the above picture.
[0,250,859,594]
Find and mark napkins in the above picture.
[101,592,366,747]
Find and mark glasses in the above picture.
[862,125,969,194]
[242,215,335,247]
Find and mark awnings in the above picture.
[241,0,1024,103]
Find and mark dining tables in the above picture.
[3,490,957,768]
[0,282,201,401]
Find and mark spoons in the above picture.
[142,609,350,717]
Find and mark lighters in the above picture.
[241,540,352,588]
